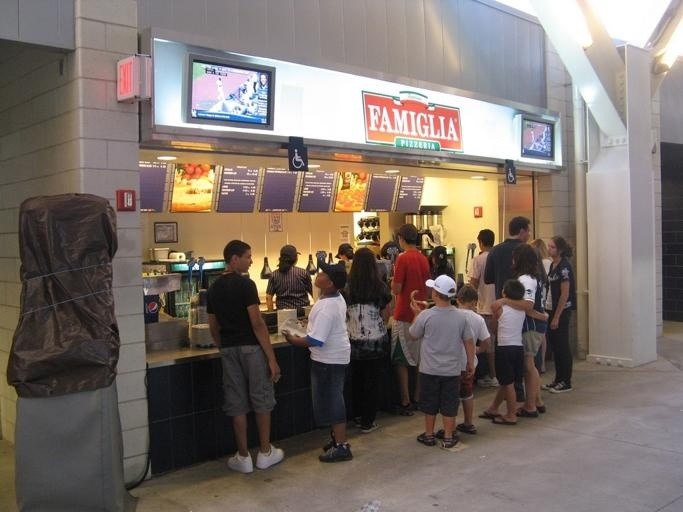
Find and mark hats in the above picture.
[318,262,347,289]
[335,243,353,259]
[280,245,301,256]
[399,223,419,240]
[425,274,457,297]
[434,246,447,262]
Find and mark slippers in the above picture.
[492,416,517,425]
[478,411,501,419]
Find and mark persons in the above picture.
[281,260,353,463]
[389,223,431,417]
[408,274,475,449]
[265,244,314,318]
[342,248,392,434]
[530,238,553,373]
[467,229,500,388]
[216,77,224,99]
[490,242,546,417]
[259,74,267,89]
[530,129,535,141]
[206,239,285,474]
[477,278,549,425]
[381,241,400,272]
[436,284,491,438]
[430,246,455,282]
[335,243,355,294]
[541,236,578,394]
[484,215,545,401]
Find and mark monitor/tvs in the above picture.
[517,114,556,160]
[185,53,276,131]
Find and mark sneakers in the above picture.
[477,374,498,387]
[323,431,336,452]
[549,379,572,393]
[353,416,362,427]
[256,443,284,470]
[540,379,561,390]
[412,399,422,411]
[417,432,436,446]
[537,405,546,413]
[388,400,413,416]
[359,422,380,434]
[457,423,476,434]
[227,449,253,474]
[319,442,353,463]
[442,434,458,448]
[434,429,457,439]
[516,407,539,417]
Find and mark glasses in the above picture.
[549,245,557,249]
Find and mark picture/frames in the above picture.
[269,213,283,232]
[153,221,178,244]
